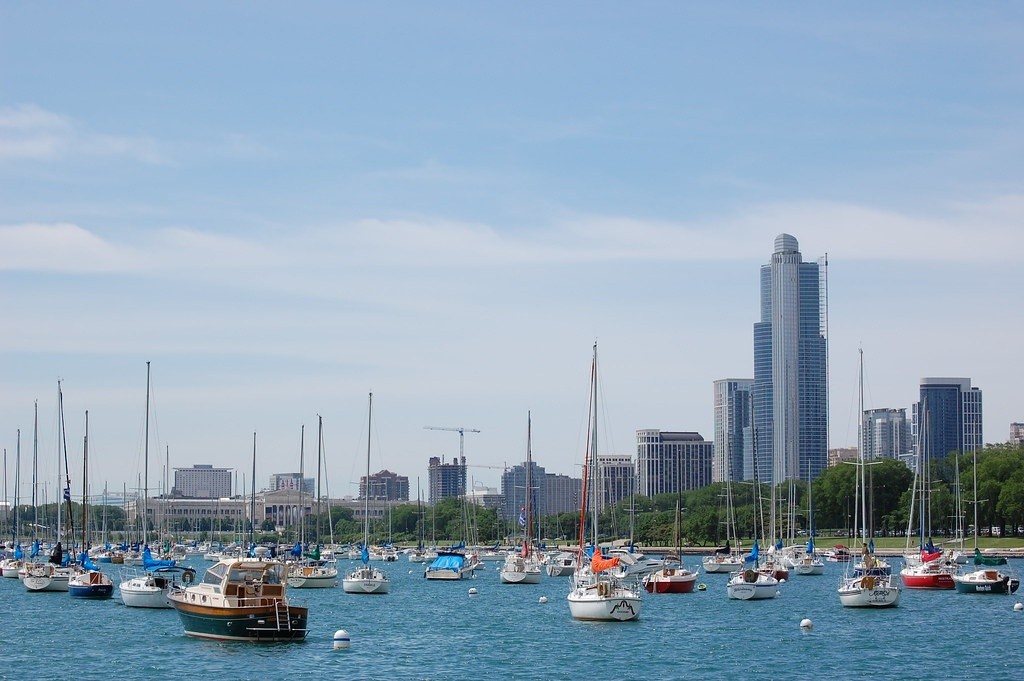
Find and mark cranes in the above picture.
[423,425,482,464]
[473,460,512,472]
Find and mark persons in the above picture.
[862,543,868,554]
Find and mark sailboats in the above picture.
[66,410,116,601]
[165,428,312,645]
[1,370,1024,594]
[342,391,390,594]
[568,333,644,622]
[837,339,905,609]
[726,391,779,601]
[117,359,198,611]
[640,446,699,594]
